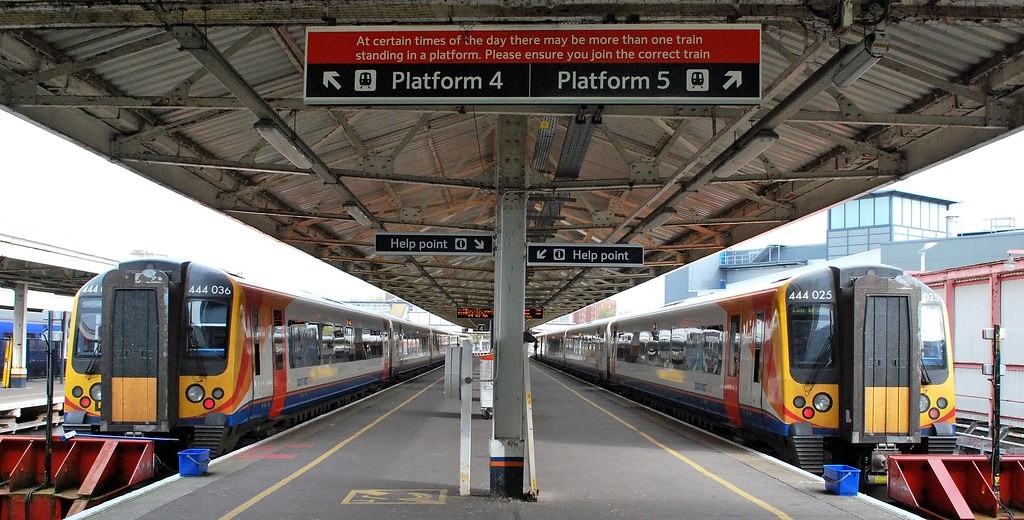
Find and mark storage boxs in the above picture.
[823,465,862,496]
[177,449,211,476]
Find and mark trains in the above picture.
[0,304,71,381]
[63,258,462,456]
[528,264,957,485]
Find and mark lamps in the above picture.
[342,201,373,226]
[404,259,458,309]
[253,108,314,170]
[831,31,892,88]
[543,267,602,309]
[711,129,780,179]
[643,207,678,231]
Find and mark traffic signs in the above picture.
[526,242,645,268]
[375,232,493,255]
